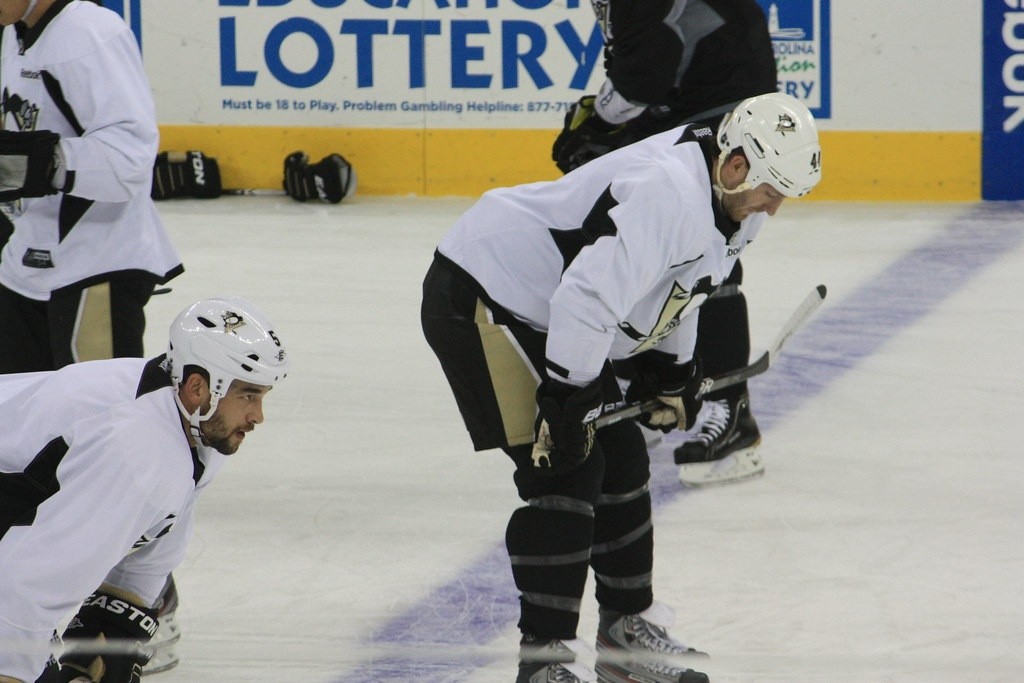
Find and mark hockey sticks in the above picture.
[531,284,828,469]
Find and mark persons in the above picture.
[550,0,777,485]
[0,298,290,683]
[420,92,823,683]
[0,0,179,675]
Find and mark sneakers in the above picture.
[136,571,181,676]
[672,389,767,488]
[515,660,590,683]
[594,606,713,683]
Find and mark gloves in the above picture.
[34,582,159,683]
[529,378,604,473]
[551,94,626,162]
[284,152,354,206]
[625,372,686,434]
[150,150,221,202]
[0,128,62,203]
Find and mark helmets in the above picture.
[167,296,289,450]
[715,92,823,198]
